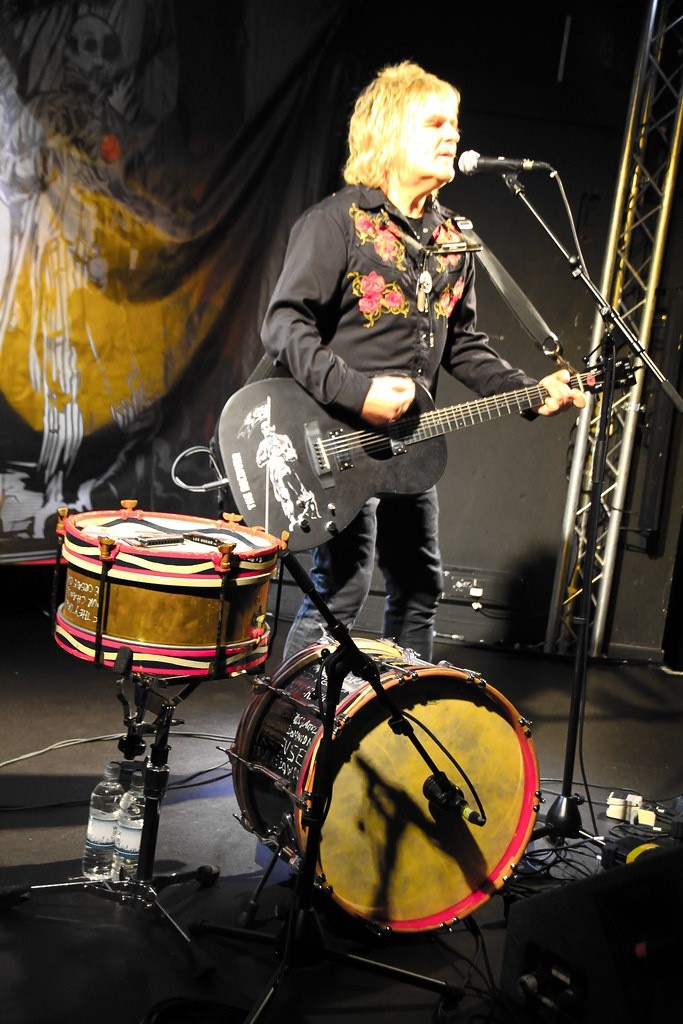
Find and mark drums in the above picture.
[53,498,292,677]
[228,635,545,934]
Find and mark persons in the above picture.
[243,61,586,663]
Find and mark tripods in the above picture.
[0,546,464,1024]
[499,172,683,851]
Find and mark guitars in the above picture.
[210,352,639,553]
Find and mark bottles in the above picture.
[111,771,145,881]
[83,762,124,881]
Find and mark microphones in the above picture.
[459,150,550,175]
[422,774,483,826]
